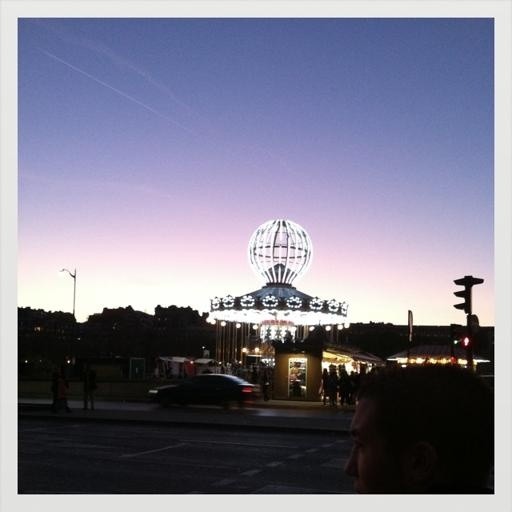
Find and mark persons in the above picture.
[321,368,493,493]
[50,365,73,413]
[78,361,99,411]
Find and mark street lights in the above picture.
[59,267,77,315]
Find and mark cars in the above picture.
[151,372,261,409]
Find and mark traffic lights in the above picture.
[461,335,472,348]
[453,275,485,314]
